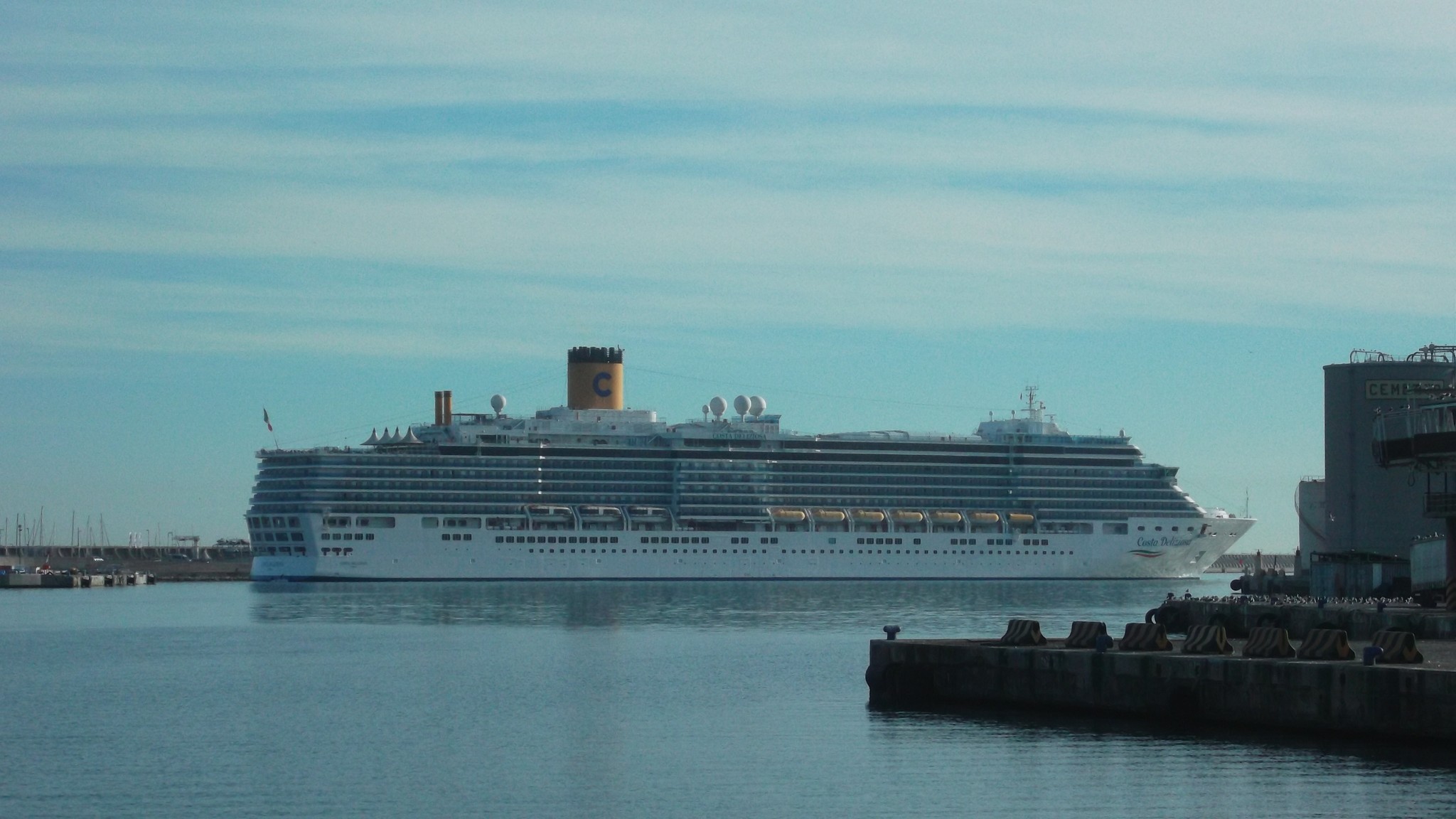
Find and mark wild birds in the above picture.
[1374,382,1455,413]
[1163,588,1420,604]
[1352,342,1456,364]
[1330,515,1336,521]
[1415,530,1446,541]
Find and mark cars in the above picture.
[91,556,104,562]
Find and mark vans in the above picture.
[167,554,192,563]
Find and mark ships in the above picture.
[242,346,1258,587]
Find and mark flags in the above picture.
[263,409,272,431]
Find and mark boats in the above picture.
[526,505,573,523]
[1008,513,1034,525]
[627,505,669,523]
[850,510,885,524]
[890,511,924,524]
[929,512,961,523]
[579,505,621,524]
[966,512,999,524]
[768,507,805,522]
[811,509,845,523]
